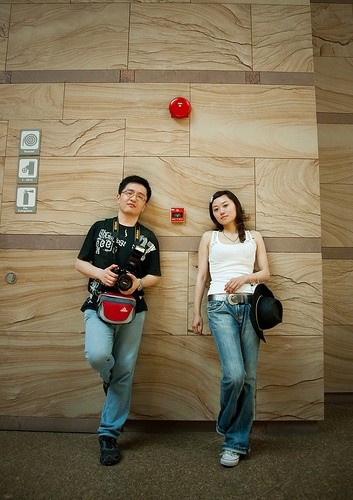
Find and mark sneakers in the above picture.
[219,450,240,466]
[102,380,110,395]
[97,435,120,466]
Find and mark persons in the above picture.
[190,190,271,468]
[73,175,161,467]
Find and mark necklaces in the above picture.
[223,230,239,242]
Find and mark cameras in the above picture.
[113,267,133,291]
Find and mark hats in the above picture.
[249,282,283,343]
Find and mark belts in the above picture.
[207,294,252,306]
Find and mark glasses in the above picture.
[118,190,147,202]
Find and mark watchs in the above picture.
[136,279,142,292]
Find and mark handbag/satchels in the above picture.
[97,291,137,325]
